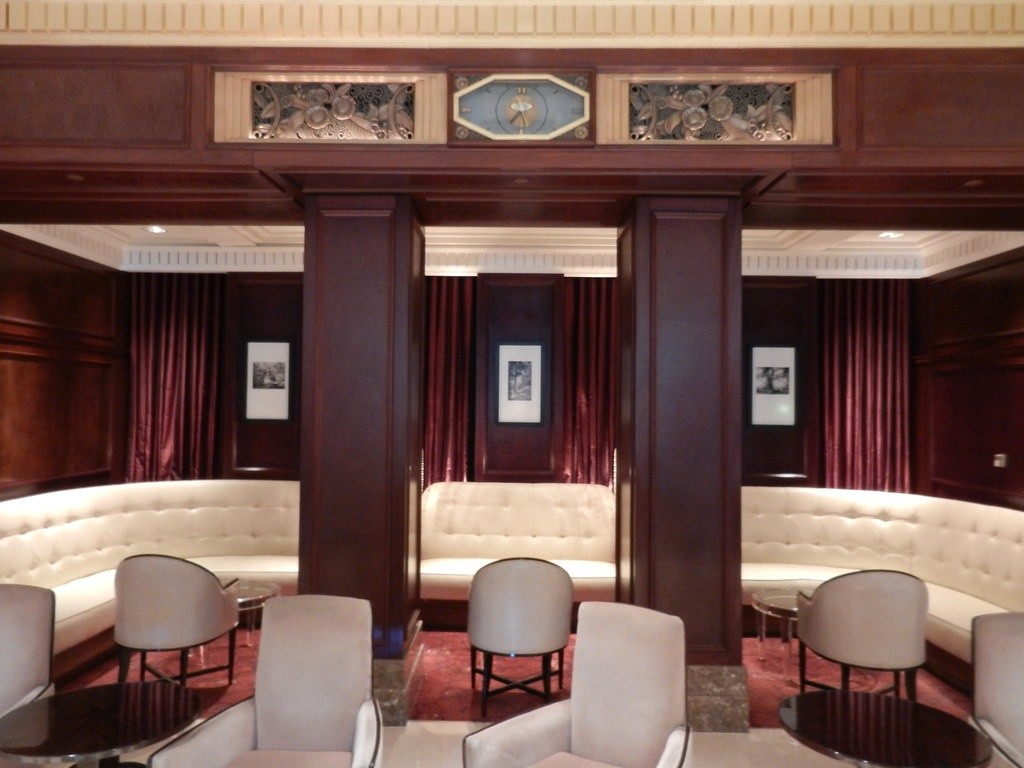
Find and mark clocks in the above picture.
[446,66,597,149]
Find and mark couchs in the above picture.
[741,486,1024,698]
[0,479,300,681]
[420,482,616,627]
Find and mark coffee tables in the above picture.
[0,682,202,768]
[751,587,815,682]
[188,578,282,668]
[776,691,993,768]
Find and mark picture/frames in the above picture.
[239,335,296,425]
[494,339,547,427]
[744,340,802,431]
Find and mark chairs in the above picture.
[0,584,62,768]
[147,595,383,768]
[115,553,239,685]
[461,601,693,768]
[966,612,1024,768]
[467,557,575,716]
[796,569,929,701]
